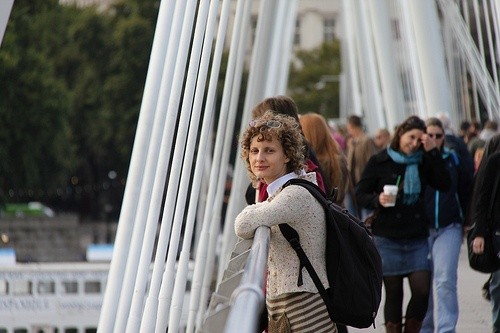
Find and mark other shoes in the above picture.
[403,317,423,333]
[384,320,402,333]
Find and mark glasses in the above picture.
[428,133,444,139]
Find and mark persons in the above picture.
[234,96,500,333]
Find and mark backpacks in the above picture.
[278,178,383,329]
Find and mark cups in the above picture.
[383,185,398,207]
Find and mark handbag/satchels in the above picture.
[467,227,500,273]
